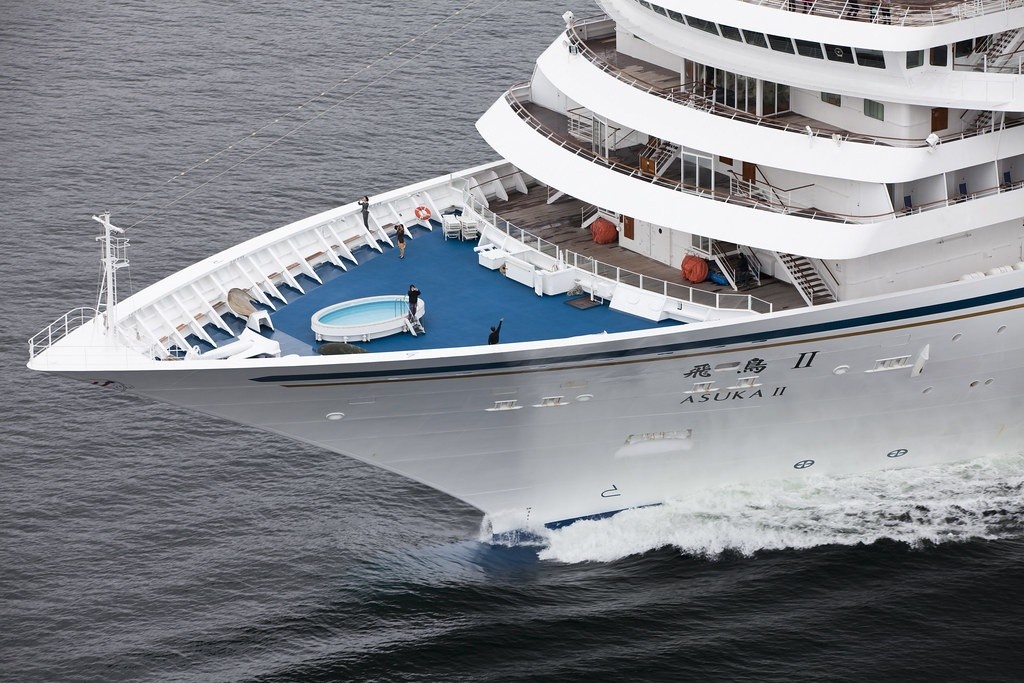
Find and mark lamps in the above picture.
[806,126,813,142]
[832,133,842,148]
[569,45,579,56]
[925,133,939,153]
[563,40,571,49]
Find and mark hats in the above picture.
[491,326,496,331]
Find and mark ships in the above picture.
[24,2,1024,531]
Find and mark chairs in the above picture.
[441,214,479,242]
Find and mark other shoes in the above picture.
[398,254,404,259]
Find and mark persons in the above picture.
[394,223,406,259]
[488,318,504,345]
[789,0,796,12]
[358,195,370,229]
[846,0,860,19]
[880,0,891,25]
[737,252,748,287]
[407,285,421,321]
[802,0,815,15]
[868,0,879,22]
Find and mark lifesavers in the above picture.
[415,207,432,220]
